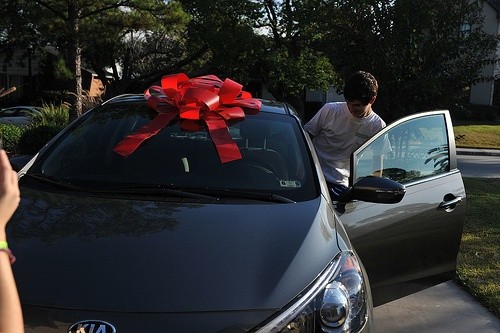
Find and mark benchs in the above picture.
[170,136,245,179]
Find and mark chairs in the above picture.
[225,119,289,185]
[119,117,187,176]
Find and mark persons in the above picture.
[0,148,24,333]
[304,71,393,200]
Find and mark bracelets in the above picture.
[0,248,16,264]
[0,241,8,249]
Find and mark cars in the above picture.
[0,107,49,126]
[6,91,468,332]
